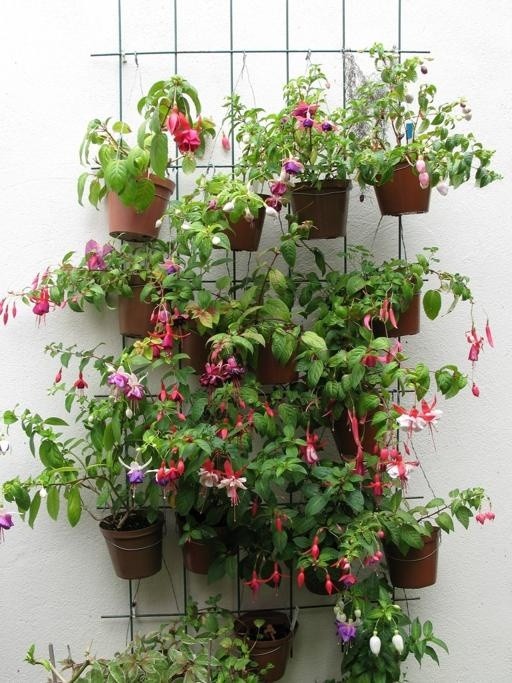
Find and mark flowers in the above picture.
[1,33,505,681]
